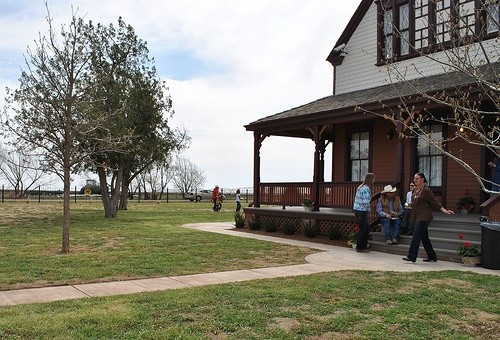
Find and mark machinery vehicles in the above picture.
[79,178,115,195]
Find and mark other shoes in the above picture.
[406,231,413,235]
[357,248,369,252]
[387,239,392,244]
[392,239,398,245]
[400,228,408,234]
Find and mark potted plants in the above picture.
[303,220,320,238]
[302,200,313,212]
[328,224,341,241]
[234,212,246,228]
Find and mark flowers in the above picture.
[456,189,475,212]
[347,226,360,243]
[456,233,481,256]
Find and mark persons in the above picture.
[353,174,375,253]
[402,172,454,263]
[400,180,417,236]
[236,189,244,212]
[376,184,404,245]
[488,146,500,196]
[212,187,222,212]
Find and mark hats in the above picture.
[381,185,397,194]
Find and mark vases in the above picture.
[463,256,480,267]
[461,210,469,215]
[348,240,357,250]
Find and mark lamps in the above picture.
[386,127,394,139]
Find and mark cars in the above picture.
[184,189,228,202]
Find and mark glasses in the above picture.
[409,185,413,187]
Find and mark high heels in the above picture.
[402,256,416,262]
[423,257,437,262]
